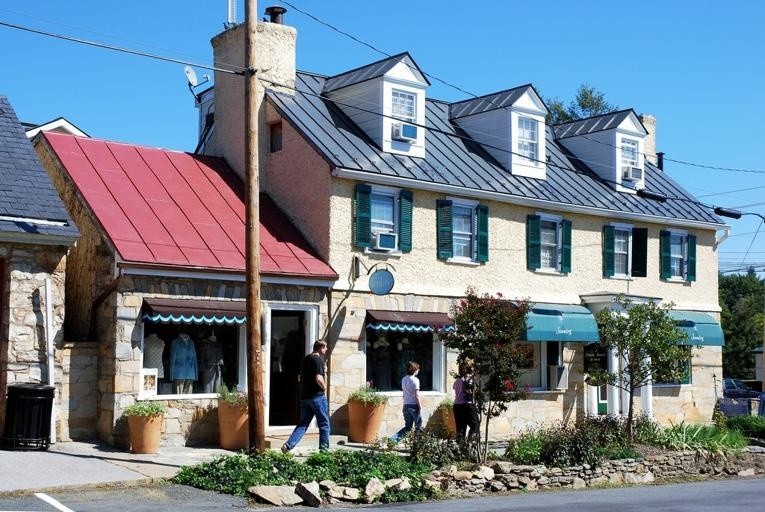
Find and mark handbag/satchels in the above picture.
[463,378,474,402]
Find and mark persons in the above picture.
[375,334,396,393]
[396,339,419,387]
[365,339,375,392]
[138,332,166,393]
[385,359,429,450]
[169,327,199,395]
[198,334,227,394]
[451,361,481,444]
[280,338,332,454]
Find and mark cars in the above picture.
[722,378,765,398]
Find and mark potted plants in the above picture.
[218,381,249,449]
[437,396,457,433]
[122,400,165,453]
[346,386,389,444]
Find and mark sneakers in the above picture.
[282,445,288,454]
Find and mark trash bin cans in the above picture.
[0,382,56,452]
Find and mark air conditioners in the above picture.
[372,232,398,251]
[393,122,418,143]
[622,165,643,182]
